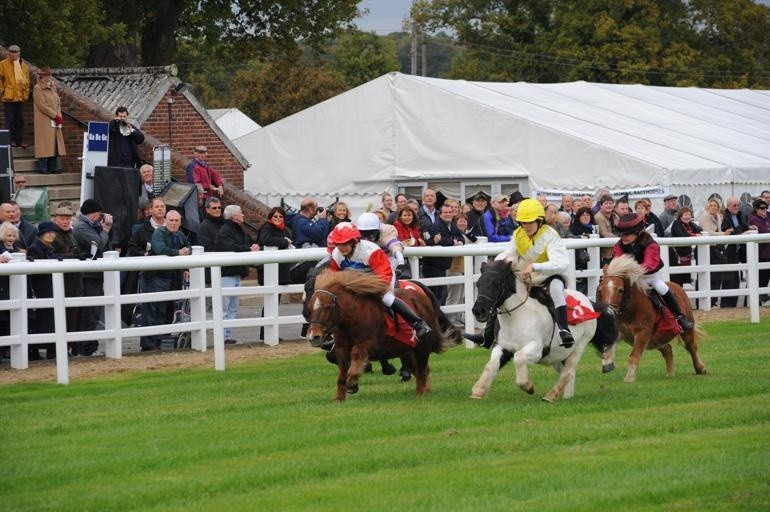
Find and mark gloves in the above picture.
[54,115,63,125]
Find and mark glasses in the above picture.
[759,206,768,209]
[211,207,221,209]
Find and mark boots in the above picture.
[391,296,432,339]
[462,312,496,349]
[662,289,692,330]
[554,305,574,348]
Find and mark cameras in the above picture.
[117,118,128,127]
[317,205,334,216]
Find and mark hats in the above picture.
[473,192,530,207]
[195,146,207,151]
[9,45,20,51]
[37,199,102,236]
[664,195,677,201]
[37,66,53,75]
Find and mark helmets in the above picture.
[615,213,643,234]
[332,213,380,244]
[516,199,545,227]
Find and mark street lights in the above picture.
[167,81,192,148]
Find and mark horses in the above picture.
[597,253,707,383]
[306,267,459,404]
[468,258,619,404]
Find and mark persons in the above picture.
[184,145,224,222]
[32,66,67,174]
[136,164,153,210]
[0,45,31,147]
[0,186,768,360]
[109,107,144,168]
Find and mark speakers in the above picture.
[92,165,141,257]
